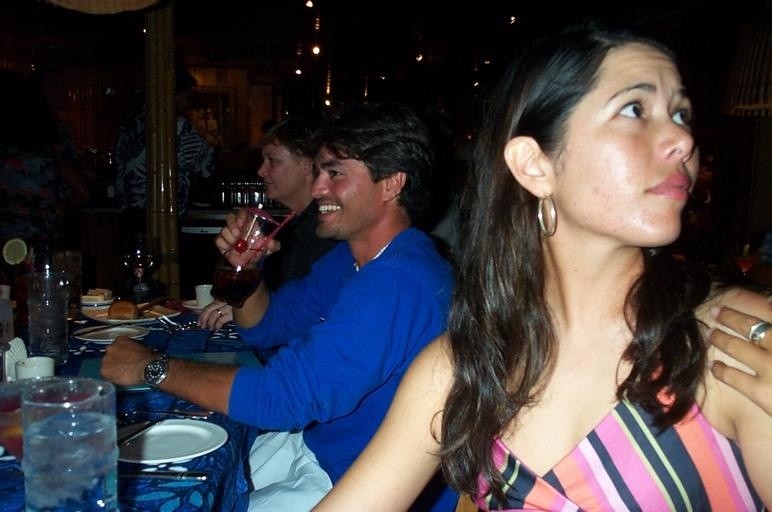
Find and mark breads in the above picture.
[80,288,112,304]
[107,300,138,320]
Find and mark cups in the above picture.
[21,376,120,512]
[0,375,63,465]
[24,264,72,370]
[13,355,57,383]
[211,211,275,309]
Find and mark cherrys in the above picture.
[234,239,249,253]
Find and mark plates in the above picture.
[1,284,236,366]
[110,418,230,467]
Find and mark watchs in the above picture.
[143,353,170,391]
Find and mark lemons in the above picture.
[3,237,27,265]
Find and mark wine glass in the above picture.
[730,240,760,291]
[120,230,157,305]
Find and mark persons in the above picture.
[113,69,231,297]
[313,19,772,511]
[0,67,90,302]
[99,98,459,512]
[195,117,340,332]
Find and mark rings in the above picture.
[751,321,771,343]
[216,309,223,316]
